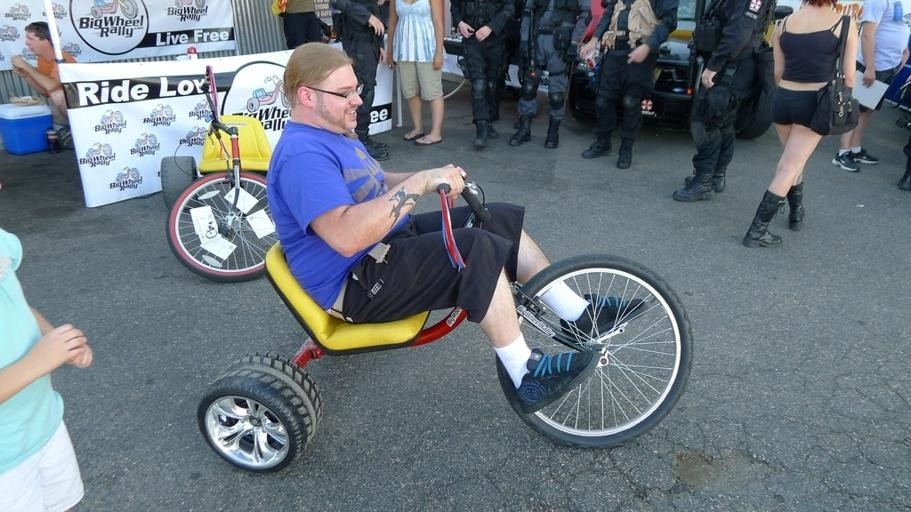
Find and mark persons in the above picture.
[450,0,677,169]
[671,1,772,200]
[829,3,911,171]
[329,0,447,161]
[745,0,857,248]
[11,22,81,127]
[268,43,650,414]
[1,229,96,512]
[284,0,322,49]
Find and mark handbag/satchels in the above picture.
[811,77,858,136]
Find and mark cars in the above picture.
[569,0,779,143]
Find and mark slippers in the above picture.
[403,128,424,141]
[415,135,442,146]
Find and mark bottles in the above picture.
[45,128,62,155]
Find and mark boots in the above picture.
[686,172,725,192]
[545,119,561,147]
[583,134,612,158]
[744,190,786,248]
[674,167,714,201]
[617,138,634,169]
[787,181,804,231]
[509,115,531,146]
[473,120,498,147]
[356,131,389,160]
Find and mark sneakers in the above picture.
[558,293,647,347]
[851,148,880,164]
[832,151,860,172]
[516,348,599,413]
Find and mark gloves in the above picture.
[519,40,530,61]
[564,42,578,65]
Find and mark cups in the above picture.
[584,50,597,70]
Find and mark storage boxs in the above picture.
[0,104,53,155]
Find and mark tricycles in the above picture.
[197,163,699,465]
[162,61,313,279]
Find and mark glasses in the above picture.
[306,83,365,100]
